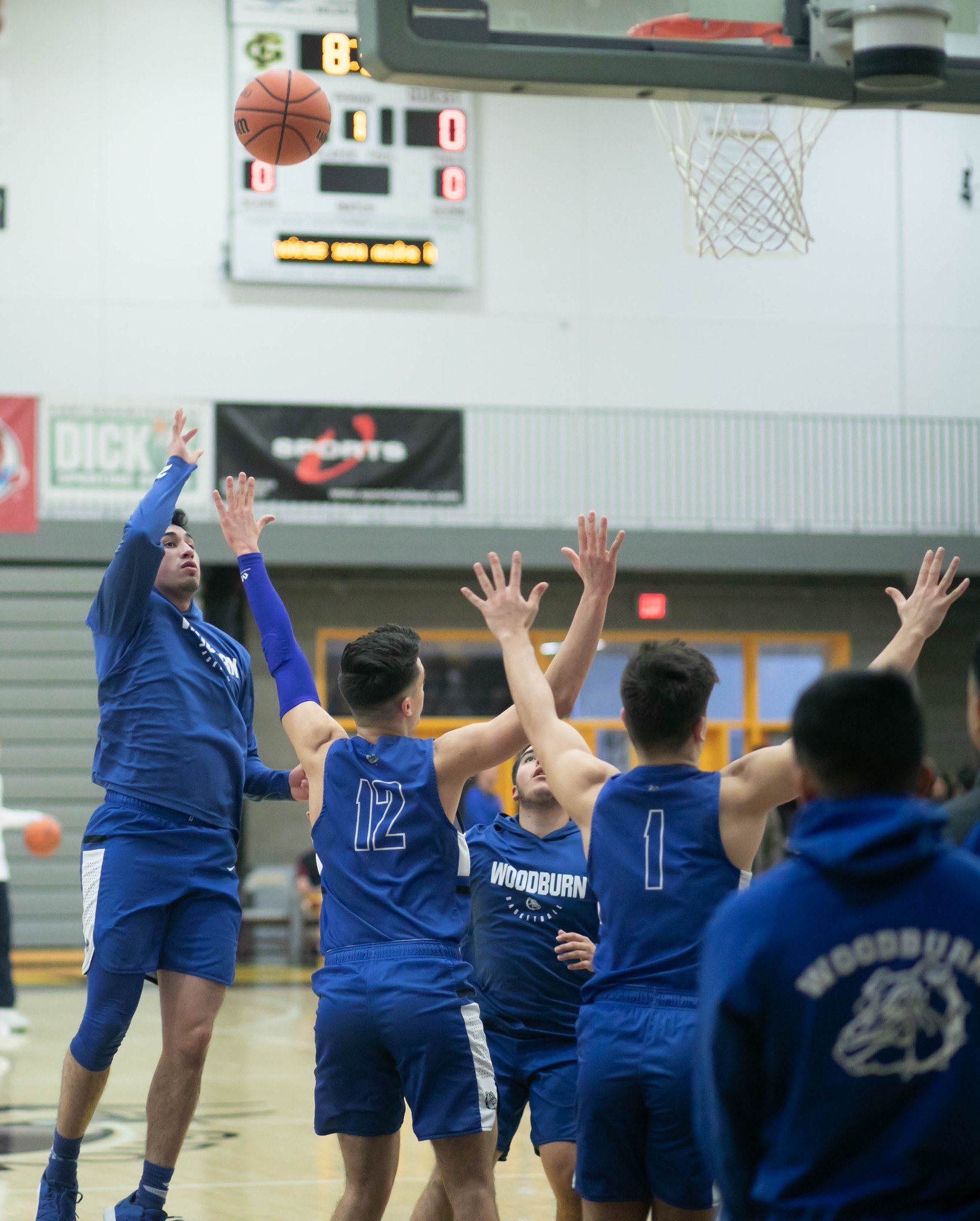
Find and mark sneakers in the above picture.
[37,1169,76,1220]
[103,1189,167,1221]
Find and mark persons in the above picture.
[37,410,309,1220]
[1,774,45,1070]
[297,547,980,1221]
[212,472,624,1220]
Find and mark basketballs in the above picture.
[231,67,332,168]
[23,817,62,860]
